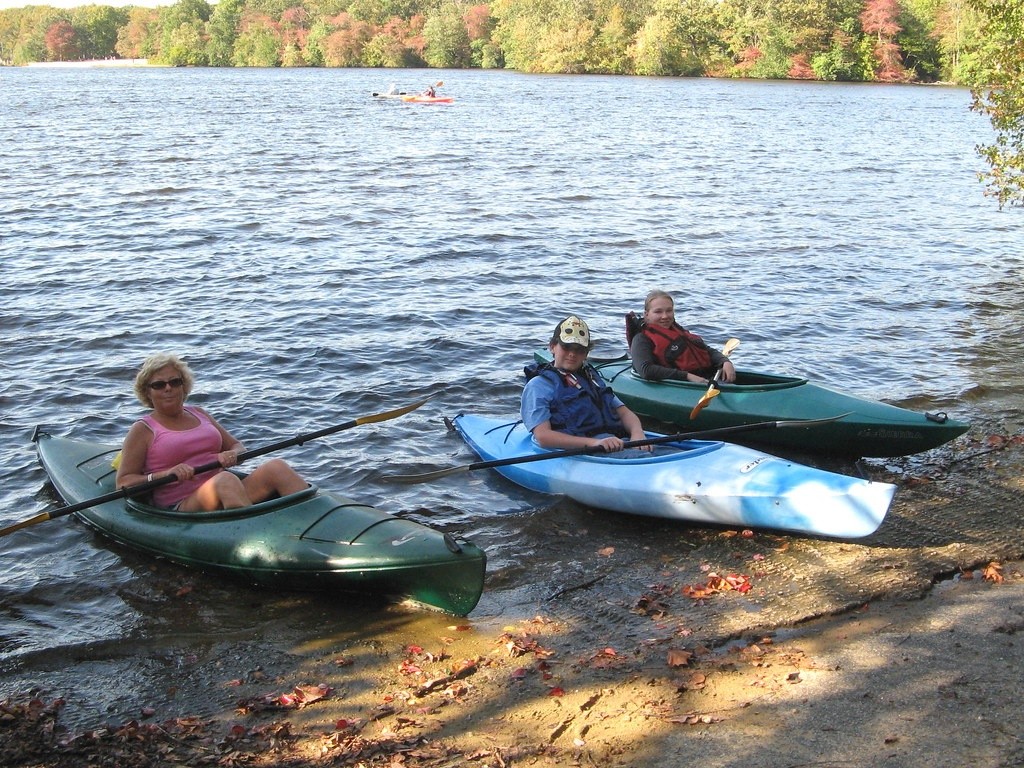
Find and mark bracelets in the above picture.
[148,473,152,482]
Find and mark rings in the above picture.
[229,455,233,459]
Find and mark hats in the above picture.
[554,316,590,347]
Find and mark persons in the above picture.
[116,354,308,512]
[520,316,652,453]
[631,290,736,384]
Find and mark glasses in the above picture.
[557,338,591,352]
[148,377,184,390]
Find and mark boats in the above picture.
[528,348,972,458]
[29,424,489,619]
[373,91,457,104]
[444,409,900,543]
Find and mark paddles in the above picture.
[0,395,430,538]
[688,336,741,422]
[379,408,859,487]
